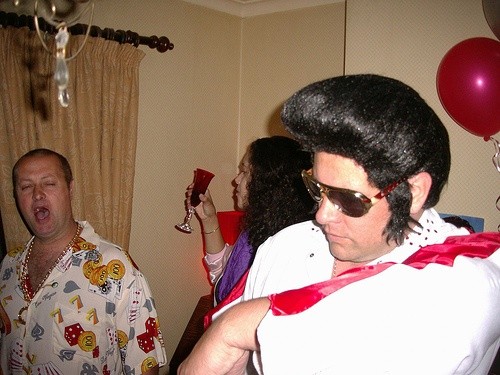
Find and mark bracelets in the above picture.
[201,223,220,235]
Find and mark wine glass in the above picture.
[175,168,215,234]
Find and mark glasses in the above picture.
[301,166,413,218]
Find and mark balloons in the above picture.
[433,37,500,140]
[481,0,500,40]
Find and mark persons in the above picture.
[1,148,168,375]
[184,136,316,309]
[173,71,499,375]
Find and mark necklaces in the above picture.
[333,259,337,278]
[19,222,80,304]
